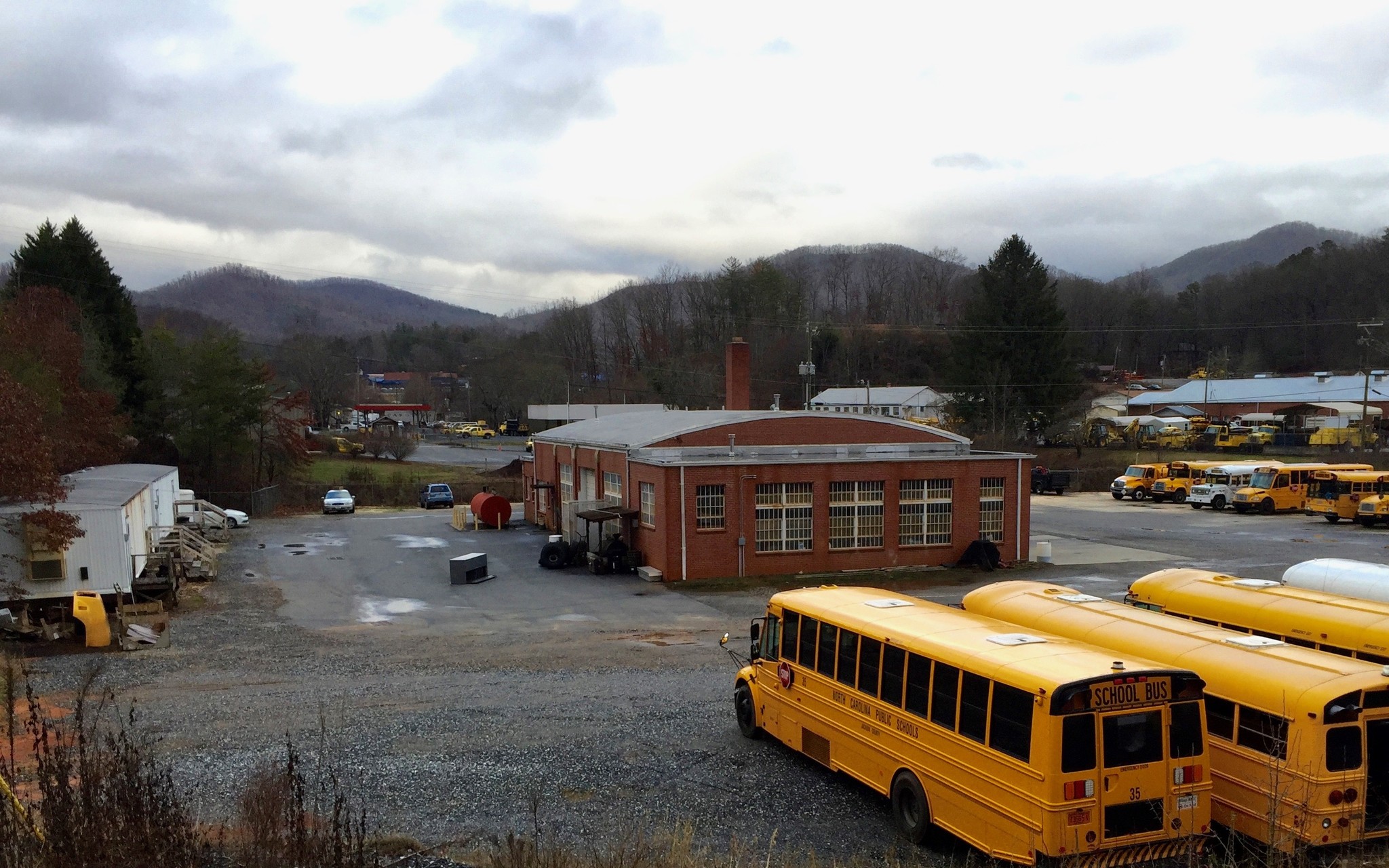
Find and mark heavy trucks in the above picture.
[498,420,529,437]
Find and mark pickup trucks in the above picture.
[426,420,496,439]
[339,421,368,432]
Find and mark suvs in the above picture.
[419,483,455,510]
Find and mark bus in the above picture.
[1109,462,1170,502]
[1123,568,1389,675]
[1232,463,1374,515]
[946,580,1389,860]
[1357,475,1389,527]
[1150,460,1284,504]
[1186,463,1270,512]
[1300,469,1389,525]
[1281,557,1389,607]
[719,584,1214,868]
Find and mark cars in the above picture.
[328,437,365,454]
[195,501,249,530]
[321,490,356,515]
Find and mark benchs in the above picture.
[637,566,663,582]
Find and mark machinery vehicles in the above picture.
[1053,412,1283,455]
[1308,425,1382,454]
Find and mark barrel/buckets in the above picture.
[1037,542,1051,557]
[549,535,563,543]
